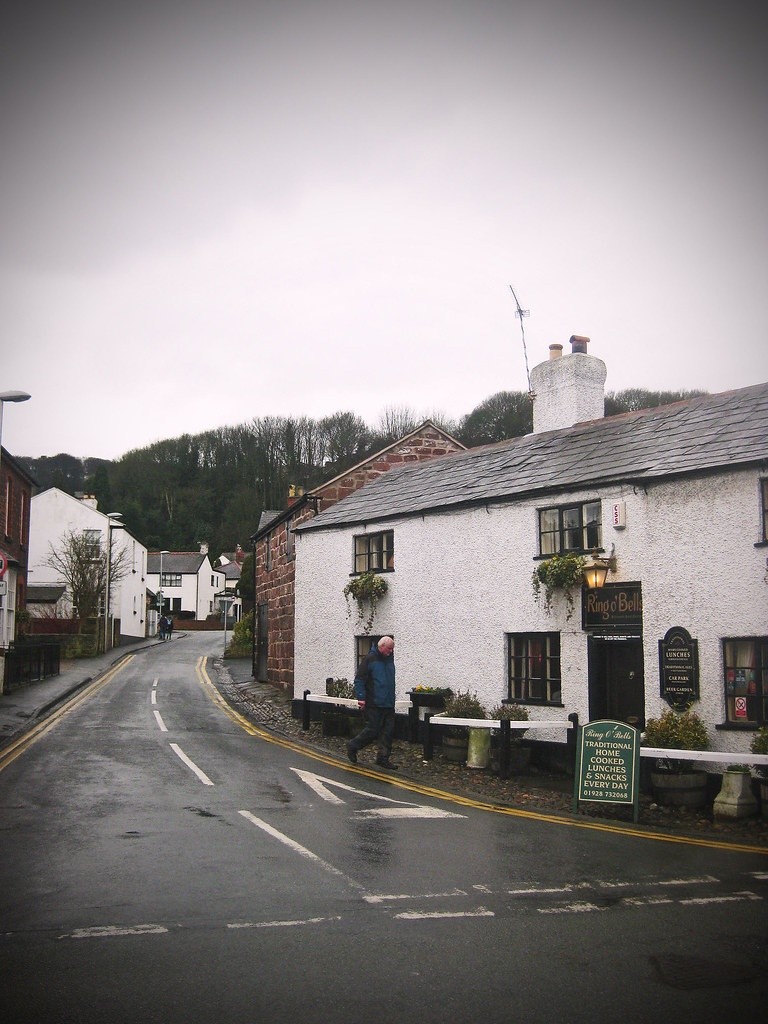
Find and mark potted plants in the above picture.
[436,687,487,762]
[640,702,710,808]
[486,702,532,774]
[343,569,389,637]
[531,553,586,621]
[748,726,768,817]
[322,678,365,737]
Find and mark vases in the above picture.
[404,692,442,709]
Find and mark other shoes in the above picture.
[375,759,398,770]
[346,740,357,763]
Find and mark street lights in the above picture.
[0,390,32,447]
[105,513,123,654]
[160,551,171,618]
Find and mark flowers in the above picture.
[411,685,452,695]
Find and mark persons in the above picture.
[167,617,173,640]
[157,615,168,640]
[346,636,399,769]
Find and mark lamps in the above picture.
[582,547,617,595]
